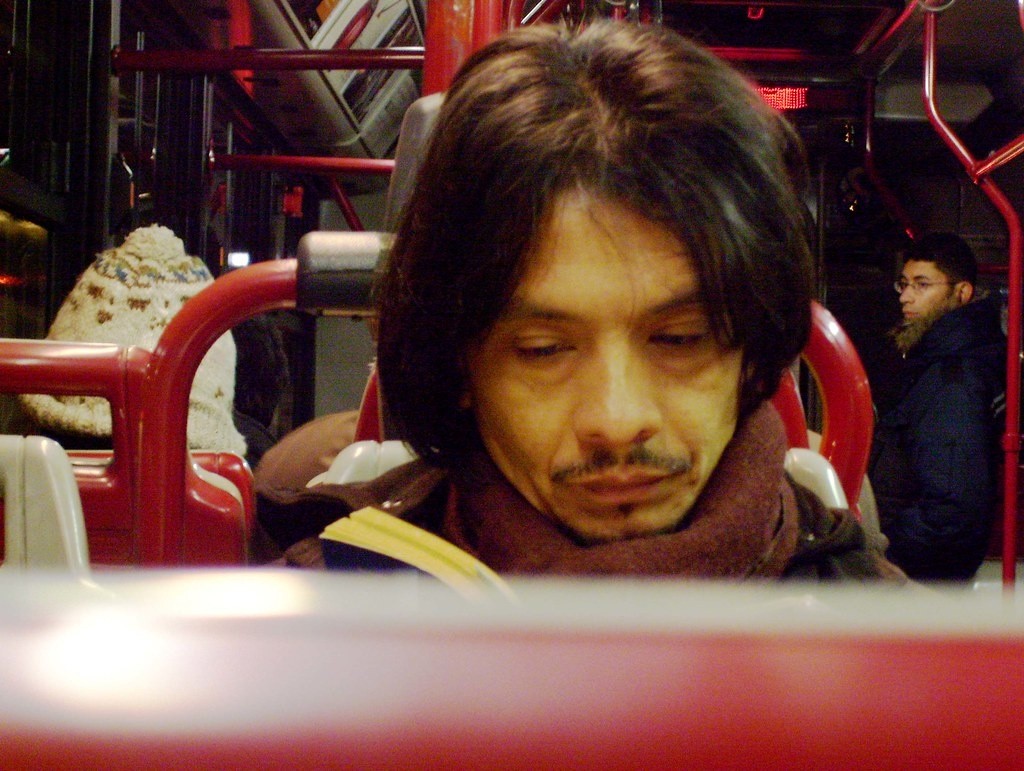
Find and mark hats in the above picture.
[19,222,249,458]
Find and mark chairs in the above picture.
[0,230,874,567]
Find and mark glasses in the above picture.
[894,281,959,294]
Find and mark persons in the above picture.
[845,228,1014,583]
[246,13,912,586]
[14,218,247,462]
